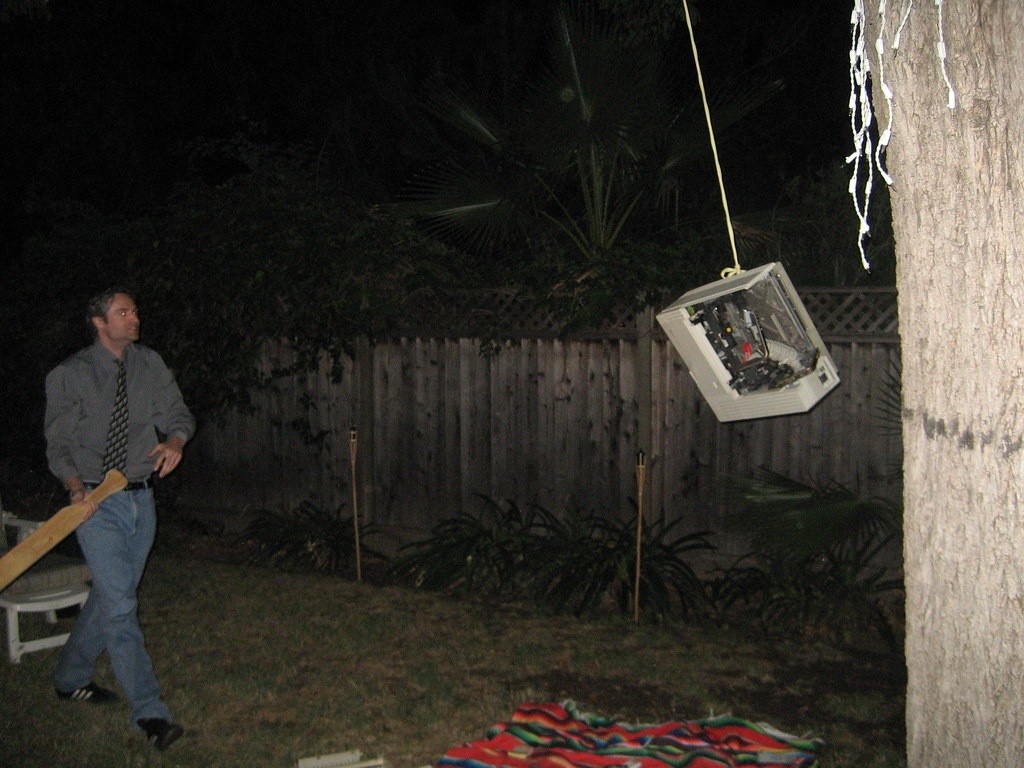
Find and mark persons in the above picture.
[45,291,196,751]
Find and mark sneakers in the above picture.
[55,681,118,705]
[136,717,184,751]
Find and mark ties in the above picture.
[101,360,129,480]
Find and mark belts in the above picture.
[82,479,151,491]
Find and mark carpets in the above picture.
[432,697,822,768]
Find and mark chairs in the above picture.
[0,517,94,667]
